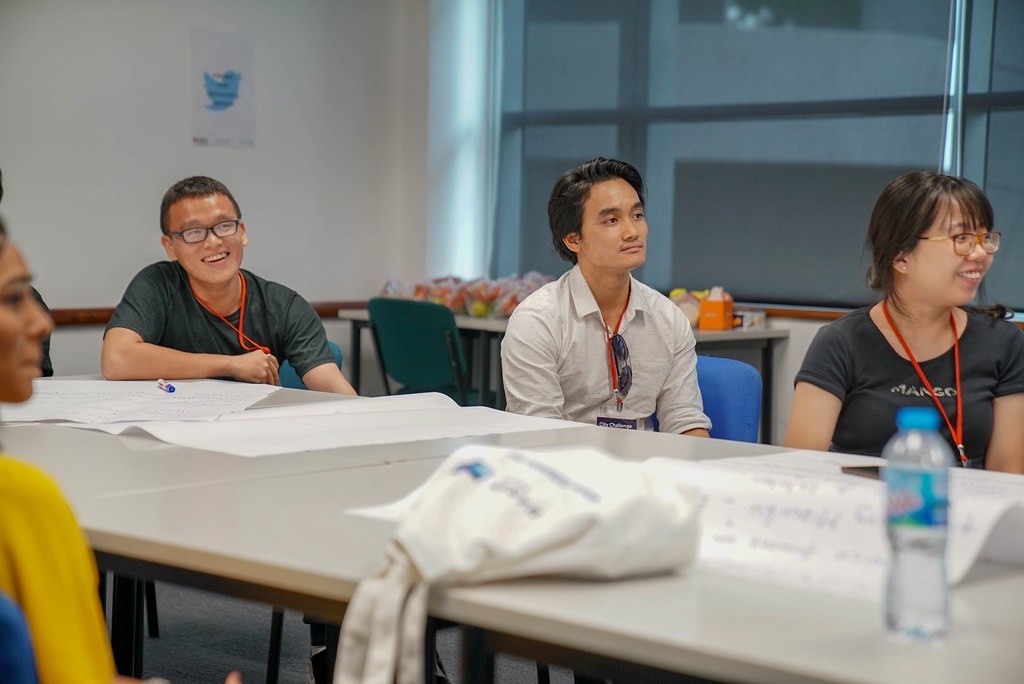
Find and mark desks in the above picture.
[0,372,1024,684]
[336,308,791,445]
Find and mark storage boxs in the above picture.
[734,311,766,331]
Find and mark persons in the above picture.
[0,176,245,684]
[497,155,716,440]
[783,168,1023,476]
[100,176,448,684]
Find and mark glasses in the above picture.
[165,218,242,244]
[919,231,1002,256]
[610,333,633,397]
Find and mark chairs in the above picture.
[367,298,507,410]
[143,341,343,641]
[537,355,765,684]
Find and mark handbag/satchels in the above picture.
[331,442,706,684]
[379,270,558,322]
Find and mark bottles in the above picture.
[879,406,957,637]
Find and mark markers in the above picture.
[156,379,175,393]
